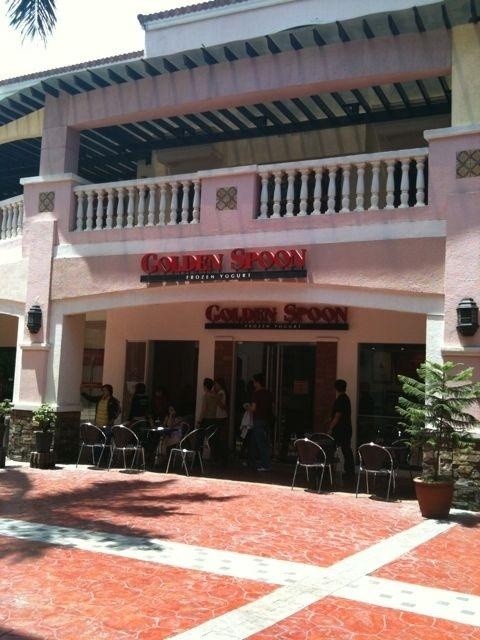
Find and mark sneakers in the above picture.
[341,487,356,493]
[256,465,273,473]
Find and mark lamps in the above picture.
[456,297,479,337]
[27,305,42,334]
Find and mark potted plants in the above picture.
[395,360,480,519]
[32,404,57,453]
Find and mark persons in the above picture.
[326,379,356,481]
[195,377,229,467]
[205,377,228,459]
[242,373,276,473]
[153,386,168,449]
[126,383,150,469]
[81,383,121,469]
[150,402,184,471]
[241,379,258,468]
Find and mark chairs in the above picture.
[77,419,219,477]
[291,432,413,502]
